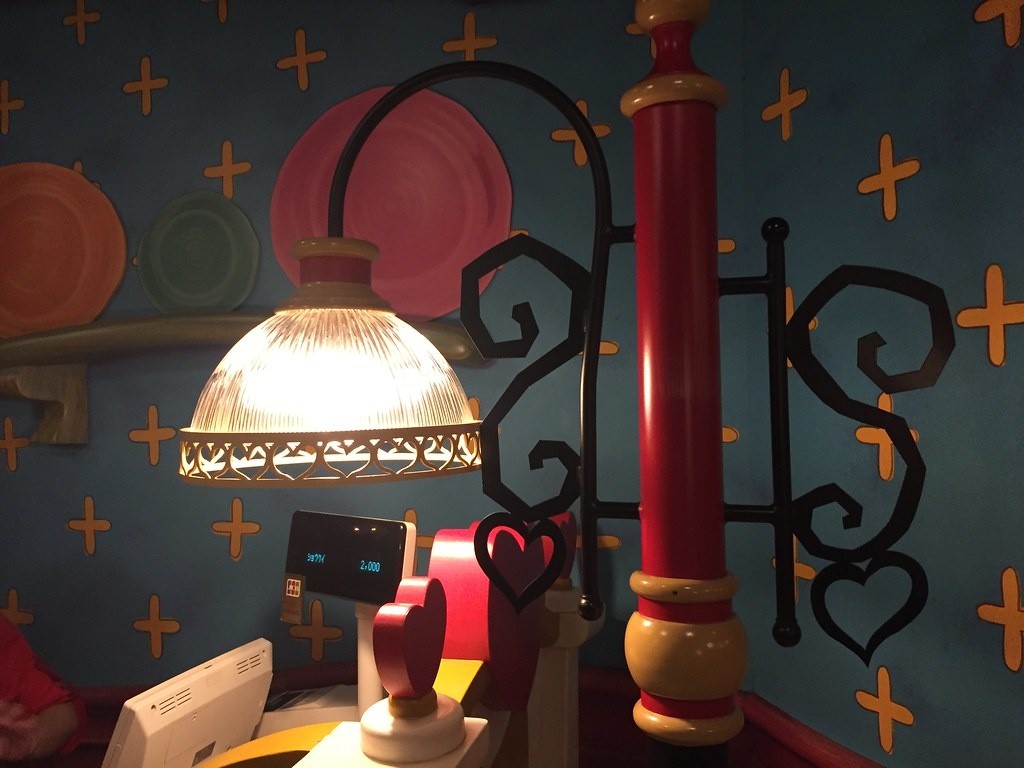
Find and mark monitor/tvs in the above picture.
[101,638,274,768]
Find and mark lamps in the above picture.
[176,60,638,622]
